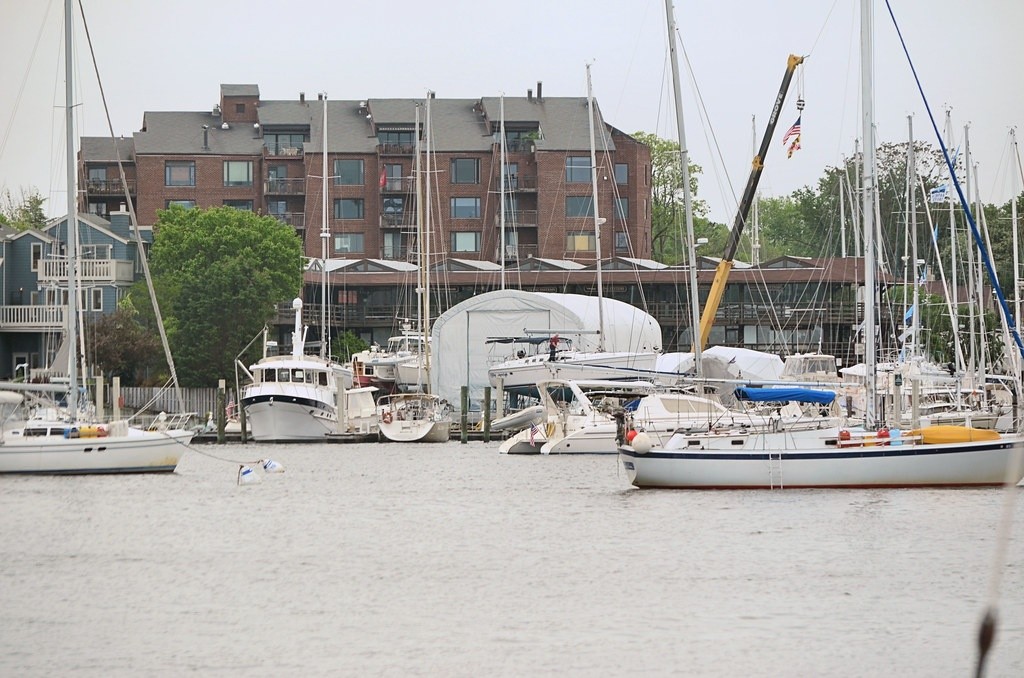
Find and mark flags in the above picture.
[899,154,957,362]
[788,134,801,159]
[783,116,801,145]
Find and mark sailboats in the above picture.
[0,0,196,474]
[236,0,1024,490]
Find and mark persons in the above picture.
[548,332,560,362]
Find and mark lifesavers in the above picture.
[382,413,393,424]
